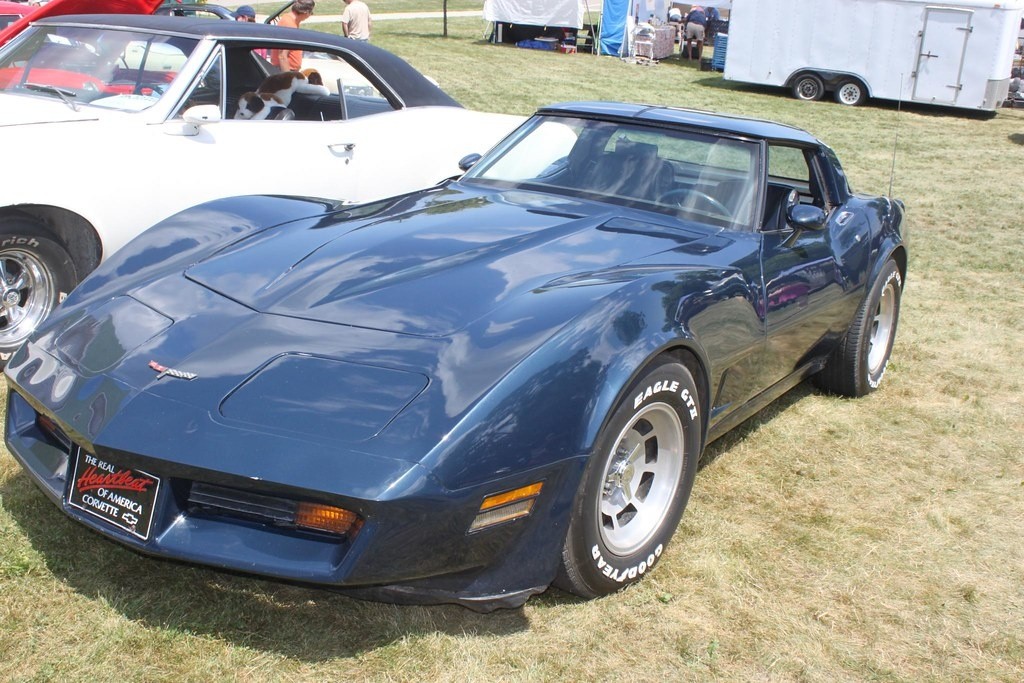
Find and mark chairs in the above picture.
[671,21,685,52]
[583,140,664,199]
[706,179,799,229]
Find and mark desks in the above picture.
[636,26,676,60]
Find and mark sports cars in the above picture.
[2,102,911,615]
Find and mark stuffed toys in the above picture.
[233,69,330,120]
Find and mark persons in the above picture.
[704,6,719,19]
[28,0,42,8]
[197,0,208,4]
[229,5,266,60]
[270,0,315,72]
[685,10,706,62]
[342,0,372,42]
[669,8,681,37]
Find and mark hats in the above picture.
[229,5,255,18]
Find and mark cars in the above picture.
[0,0,182,96]
[115,4,441,98]
[1,15,579,371]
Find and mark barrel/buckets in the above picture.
[701,57,712,72]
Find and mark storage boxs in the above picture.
[560,45,575,53]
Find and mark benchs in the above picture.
[224,86,394,120]
